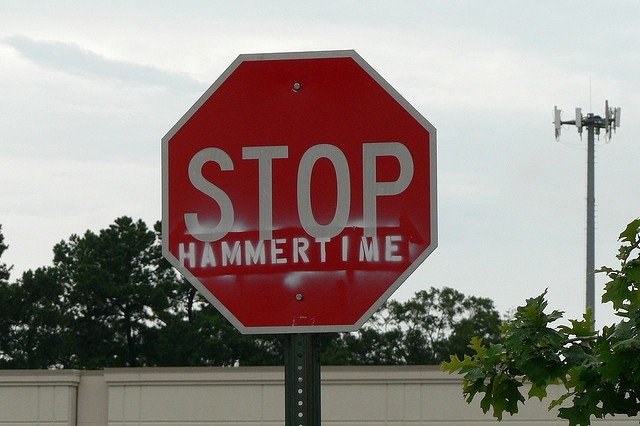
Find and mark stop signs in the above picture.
[161,48,438,335]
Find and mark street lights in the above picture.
[551,99,622,332]
